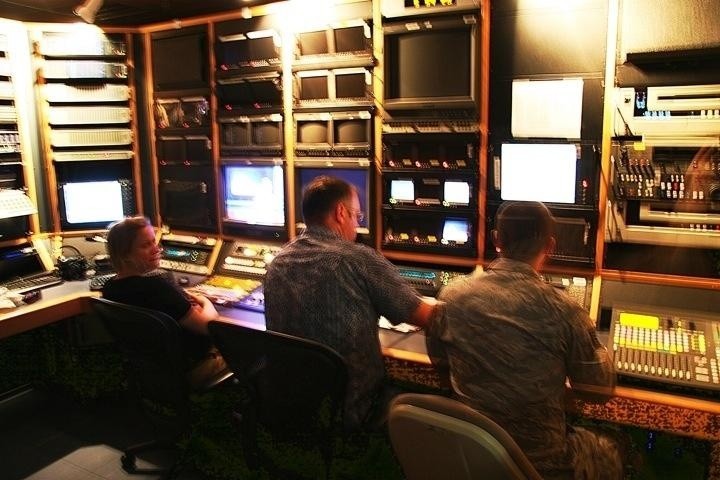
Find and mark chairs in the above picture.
[208,320,385,479]
[386,393,540,480]
[87,297,245,479]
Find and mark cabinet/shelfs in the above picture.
[1,0,719,288]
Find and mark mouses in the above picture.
[179,275,188,284]
[24,290,41,304]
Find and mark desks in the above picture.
[1,235,720,480]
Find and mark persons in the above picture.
[262,174,435,430]
[102,216,227,386]
[426,200,634,480]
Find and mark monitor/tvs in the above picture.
[149,0,603,245]
[59,182,135,231]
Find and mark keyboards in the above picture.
[90,269,173,291]
[3,272,61,294]
